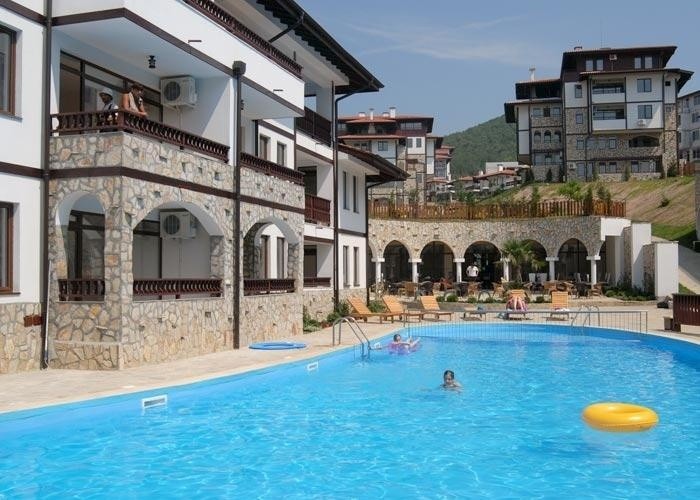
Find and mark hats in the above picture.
[98,87,114,101]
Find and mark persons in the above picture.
[118,83,148,134]
[389,334,421,354]
[506,293,526,312]
[441,370,460,391]
[95,87,119,132]
[466,263,479,282]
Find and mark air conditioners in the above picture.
[161,75,199,111]
[159,211,197,240]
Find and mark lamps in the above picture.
[147,55,156,69]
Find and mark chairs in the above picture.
[345,295,404,323]
[381,294,424,323]
[551,290,570,321]
[419,296,451,320]
[374,271,611,300]
[510,289,529,321]
[463,306,487,320]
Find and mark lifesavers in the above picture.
[388,339,422,355]
[581,402,659,432]
